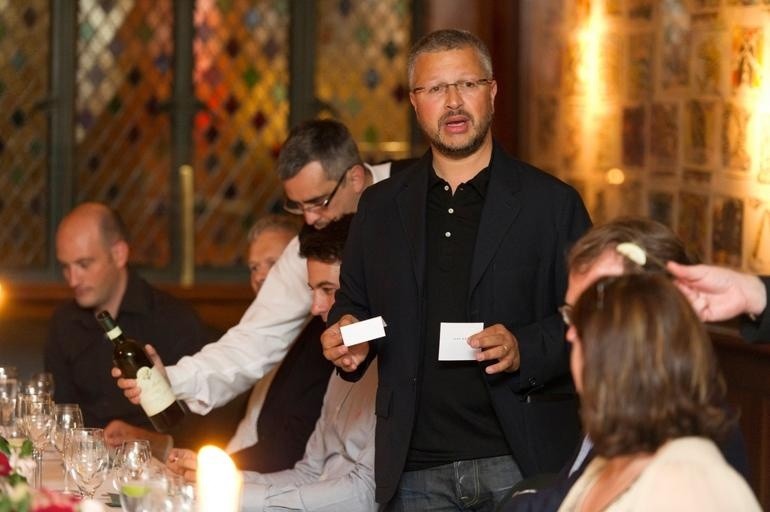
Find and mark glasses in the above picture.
[283,164,356,214]
[560,304,578,325]
[412,77,493,91]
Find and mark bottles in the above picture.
[94,310,186,435]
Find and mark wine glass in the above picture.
[65,429,111,502]
[0,364,53,490]
[51,403,84,495]
[112,439,194,512]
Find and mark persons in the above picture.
[112,121,438,416]
[320,28,594,512]
[557,217,693,357]
[218,213,385,512]
[40,201,202,465]
[223,216,303,451]
[654,235,770,331]
[552,277,766,510]
